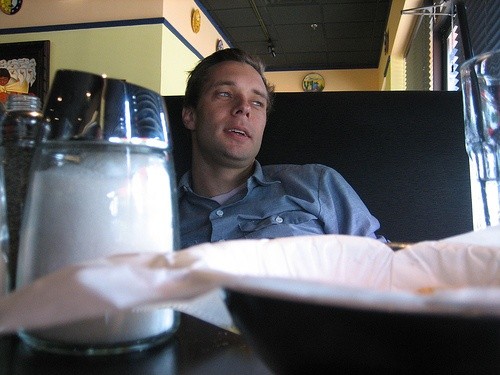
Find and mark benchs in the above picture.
[162,91,474,250]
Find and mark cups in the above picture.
[14,140,175,357]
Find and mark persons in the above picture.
[178,48,388,249]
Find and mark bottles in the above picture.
[2,96,44,210]
